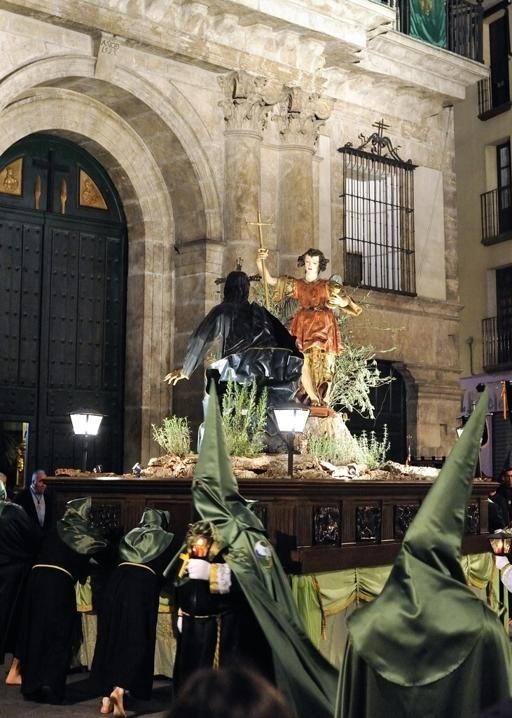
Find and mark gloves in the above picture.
[187,557,213,581]
[177,616,184,632]
[495,555,510,569]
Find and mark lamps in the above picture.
[267,400,312,477]
[65,406,109,474]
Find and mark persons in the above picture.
[333,507,512,716]
[161,667,293,718]
[481,477,512,528]
[162,458,277,691]
[20,494,100,707]
[160,269,294,456]
[15,471,53,528]
[496,466,512,527]
[254,246,361,414]
[2,478,36,688]
[91,506,177,717]
[493,554,512,595]
